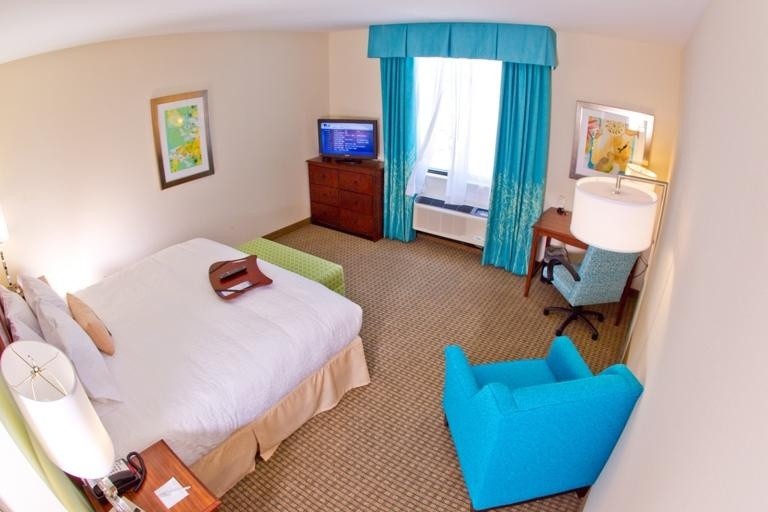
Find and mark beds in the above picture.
[23,237,363,492]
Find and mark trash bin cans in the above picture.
[540,246,567,284]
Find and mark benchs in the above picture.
[236,237,346,297]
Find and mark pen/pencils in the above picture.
[158,485,191,497]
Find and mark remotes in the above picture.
[217,264,247,280]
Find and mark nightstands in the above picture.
[80,438,222,511]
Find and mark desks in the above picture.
[524,206,642,327]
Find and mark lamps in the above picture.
[0,341,144,512]
[568,175,669,255]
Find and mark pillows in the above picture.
[0,272,123,408]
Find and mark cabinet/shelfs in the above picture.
[306,156,384,242]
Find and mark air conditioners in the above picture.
[411,194,488,249]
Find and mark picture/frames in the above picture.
[569,100,656,183]
[150,89,215,189]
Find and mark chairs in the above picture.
[440,335,644,511]
[540,244,640,341]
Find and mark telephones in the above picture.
[96,477,147,512]
[83,458,141,503]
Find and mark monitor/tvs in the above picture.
[317,119,379,165]
